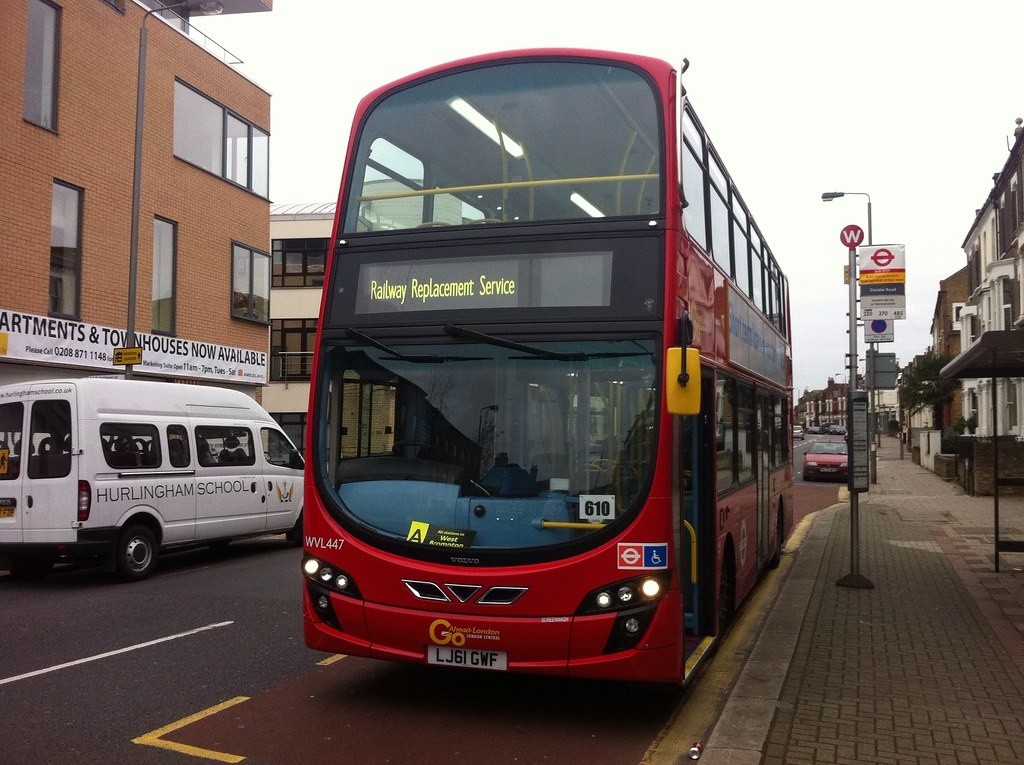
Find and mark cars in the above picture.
[828,425,847,435]
[803,439,849,481]
[793,425,804,440]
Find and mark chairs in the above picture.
[443,433,588,497]
[13,434,248,477]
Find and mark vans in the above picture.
[0,378,306,584]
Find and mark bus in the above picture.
[301,48,795,689]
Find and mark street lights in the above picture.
[821,192,873,245]
[835,373,846,390]
[125,0,224,378]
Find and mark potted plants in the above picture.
[953,414,977,435]
